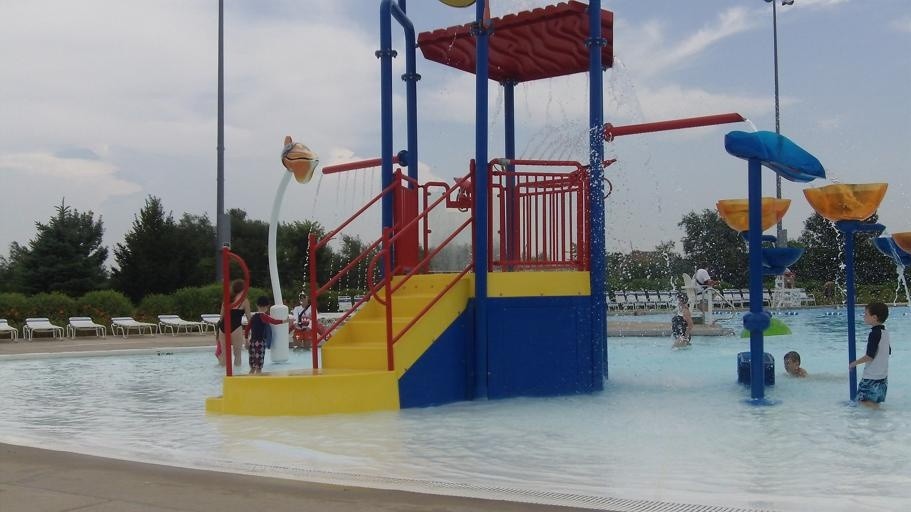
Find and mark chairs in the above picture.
[66,316,106,340]
[241,312,260,340]
[0,319,19,343]
[201,314,221,335]
[158,315,208,337]
[111,317,157,339]
[23,317,64,341]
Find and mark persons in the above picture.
[691,261,720,312]
[291,291,318,350]
[244,295,296,374]
[670,292,693,348]
[848,302,892,408]
[216,279,251,367]
[783,351,808,378]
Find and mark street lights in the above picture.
[764,0,795,276]
